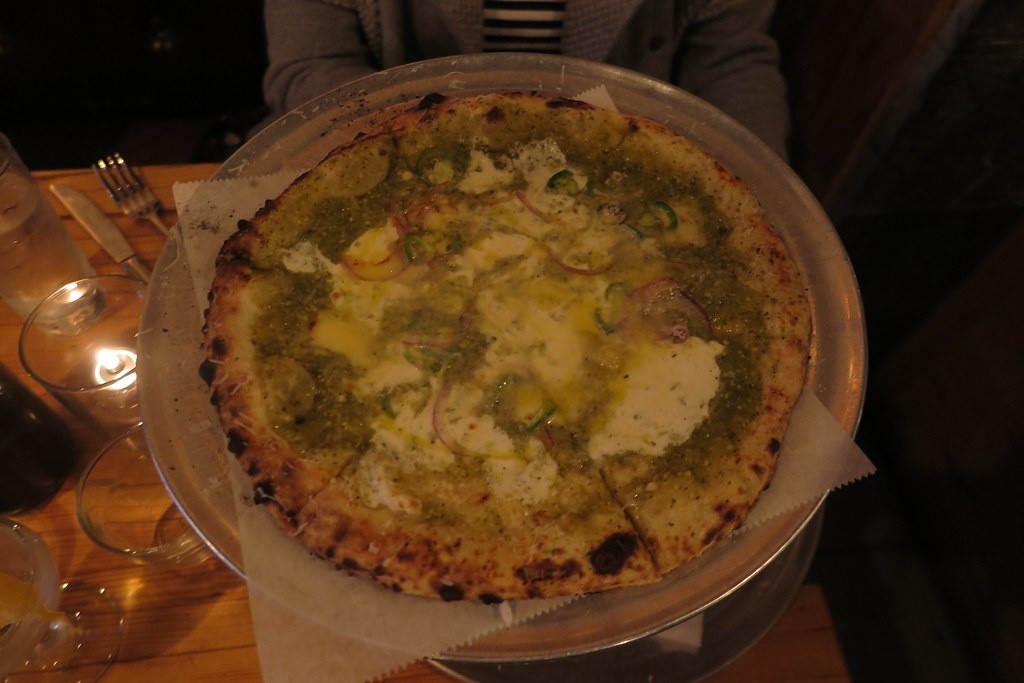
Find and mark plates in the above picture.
[133,51,867,667]
[426,498,825,682]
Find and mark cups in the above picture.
[0,132,107,333]
[73,420,212,570]
[0,519,124,683]
[18,274,147,464]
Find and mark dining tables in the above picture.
[0,165,846,683]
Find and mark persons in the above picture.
[262,1,790,168]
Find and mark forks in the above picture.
[93,152,170,235]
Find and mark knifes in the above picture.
[49,182,151,286]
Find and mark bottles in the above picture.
[0,361,76,516]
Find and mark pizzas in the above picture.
[199,93,813,602]
